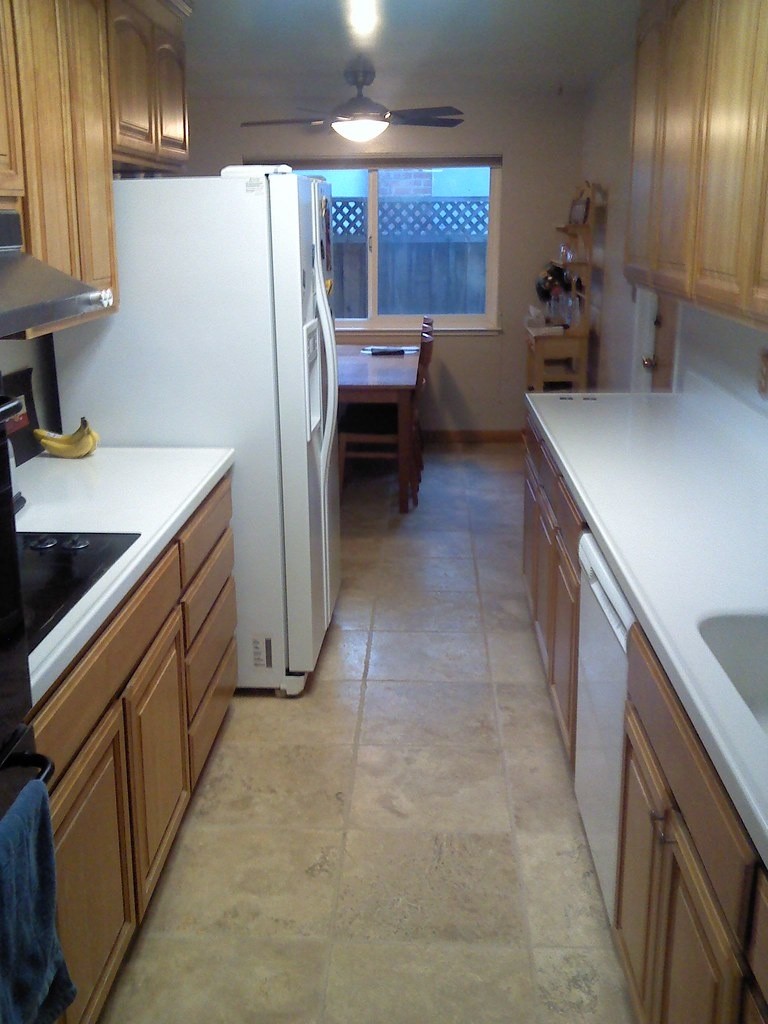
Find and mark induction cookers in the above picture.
[16,531,141,652]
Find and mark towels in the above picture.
[0,779,79,1024]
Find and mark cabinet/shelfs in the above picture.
[522,181,597,393]
[30,543,194,1024]
[622,0,768,333]
[0,0,119,341]
[172,464,239,794]
[611,620,768,1024]
[521,409,590,780]
[105,0,192,173]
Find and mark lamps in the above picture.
[332,114,391,143]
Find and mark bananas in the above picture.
[34,416,98,457]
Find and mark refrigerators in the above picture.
[52,167,346,699]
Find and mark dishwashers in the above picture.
[573,531,639,927]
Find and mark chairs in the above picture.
[339,316,434,508]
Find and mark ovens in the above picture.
[0,395,67,1024]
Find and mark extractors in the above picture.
[0,208,103,337]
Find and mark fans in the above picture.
[239,51,465,127]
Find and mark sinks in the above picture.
[696,613,768,736]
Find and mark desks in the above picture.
[335,344,421,514]
